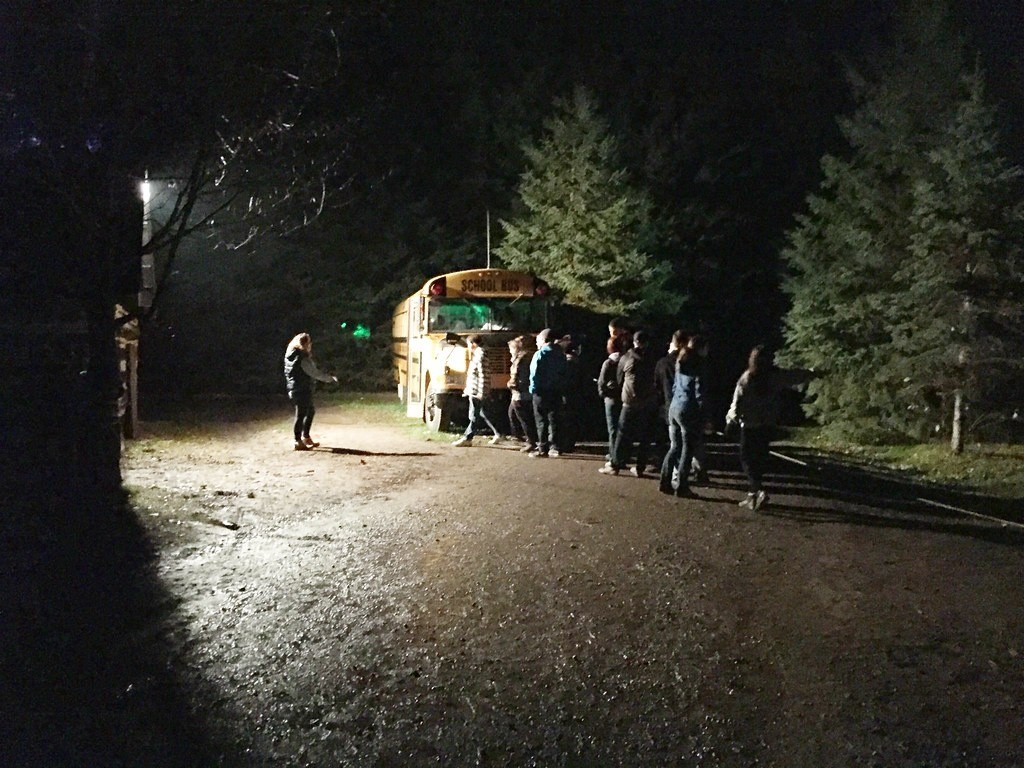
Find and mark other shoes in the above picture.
[659,483,676,495]
[604,462,612,467]
[675,490,699,499]
[302,437,320,447]
[630,467,642,477]
[753,490,770,511]
[598,466,619,476]
[488,435,507,445]
[453,437,472,446]
[528,451,549,457]
[739,493,757,510]
[295,441,313,450]
[520,444,536,453]
[549,449,562,456]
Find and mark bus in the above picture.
[390,266,554,433]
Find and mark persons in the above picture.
[727,343,783,512]
[597,316,722,499]
[453,333,507,448]
[285,332,338,450]
[507,319,588,457]
